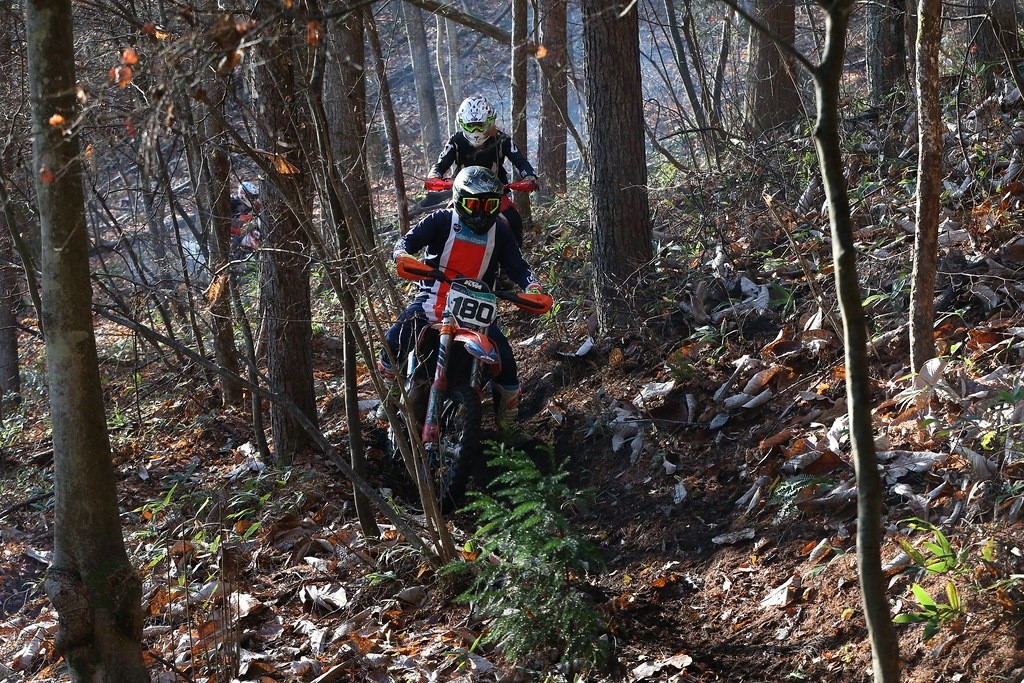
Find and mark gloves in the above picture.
[526,286,541,294]
[398,254,417,260]
[524,174,537,181]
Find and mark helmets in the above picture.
[451,166,504,235]
[456,95,499,147]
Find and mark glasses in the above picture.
[459,117,495,133]
[456,197,502,215]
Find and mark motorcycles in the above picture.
[375,257,553,513]
[231,202,262,260]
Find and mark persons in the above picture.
[427,95,538,251]
[376,166,542,445]
[229,181,261,247]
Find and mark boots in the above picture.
[496,383,527,435]
[376,348,403,419]
